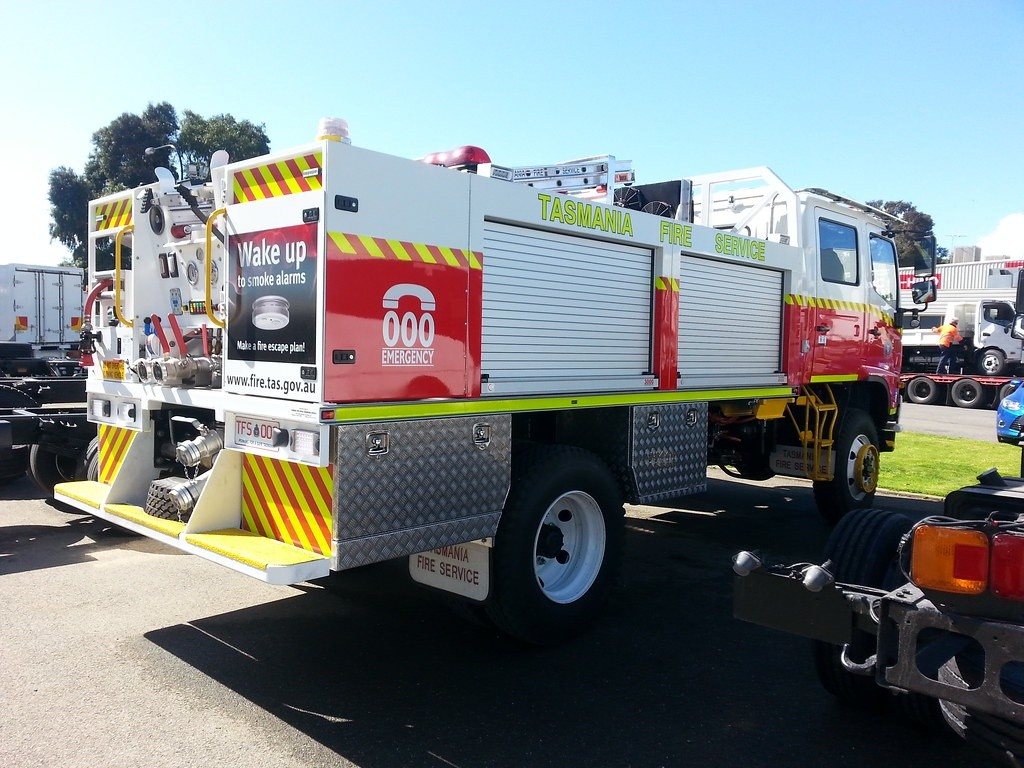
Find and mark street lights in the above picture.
[144,143,184,180]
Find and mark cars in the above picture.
[995,377,1024,445]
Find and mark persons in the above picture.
[932,317,967,375]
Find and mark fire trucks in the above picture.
[52,112,939,643]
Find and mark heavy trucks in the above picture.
[898,367,1024,410]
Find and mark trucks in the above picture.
[0,260,97,496]
[900,296,1024,376]
